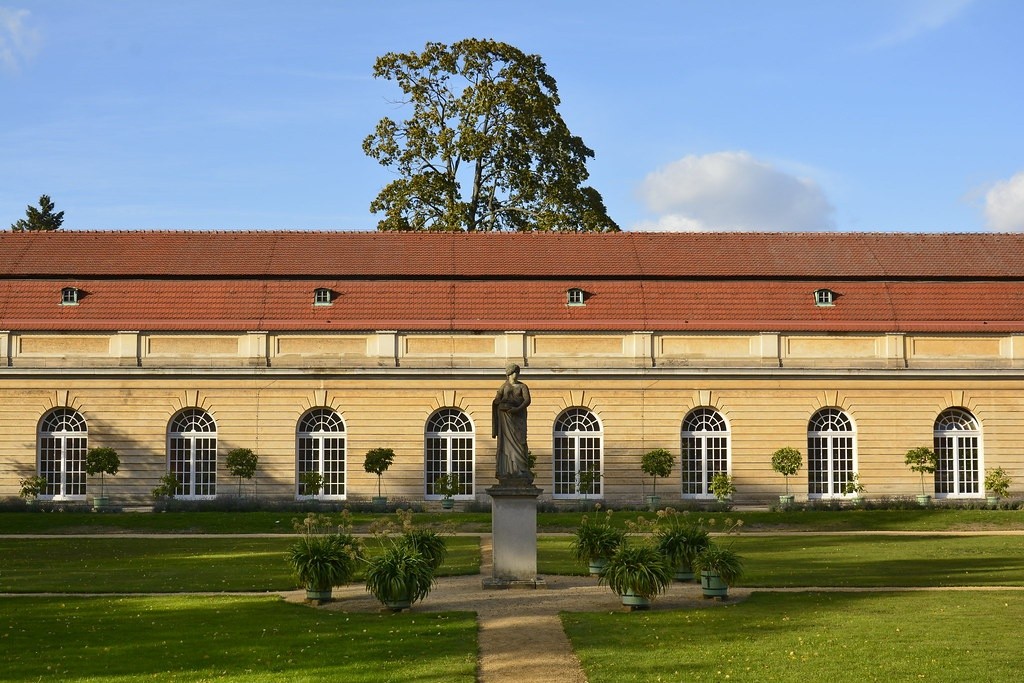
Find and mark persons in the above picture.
[490,363,534,476]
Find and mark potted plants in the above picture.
[19,476,48,512]
[283,523,364,599]
[691,538,745,598]
[85,446,121,509]
[654,511,713,578]
[708,473,738,508]
[356,527,439,608]
[598,546,675,604]
[568,522,629,574]
[904,447,938,505]
[843,472,868,506]
[435,474,463,508]
[771,446,803,509]
[575,462,606,511]
[640,448,676,513]
[300,469,327,511]
[984,466,1013,505]
[362,448,396,513]
